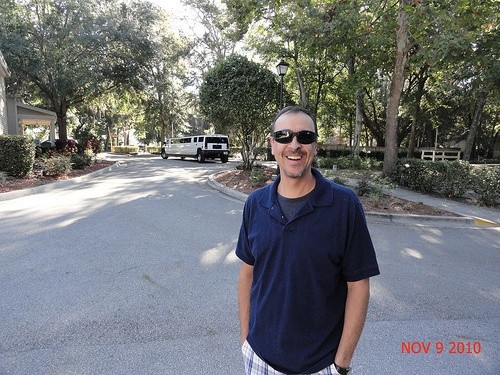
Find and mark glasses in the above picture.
[271,129,318,144]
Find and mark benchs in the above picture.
[421,147,462,161]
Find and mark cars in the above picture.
[161,134,230,163]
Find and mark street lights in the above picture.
[275,59,291,115]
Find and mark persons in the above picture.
[235,104,379,375]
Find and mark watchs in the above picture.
[334,362,351,375]
